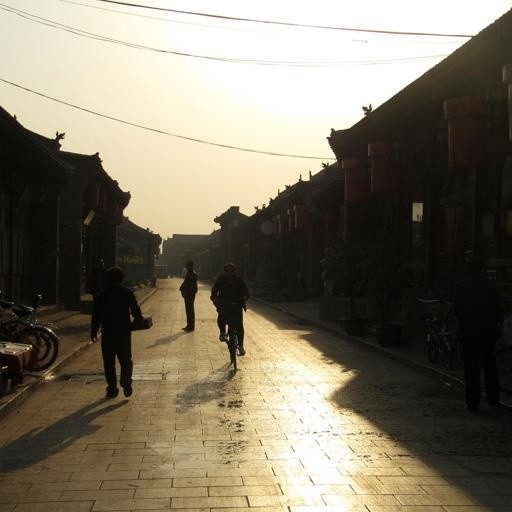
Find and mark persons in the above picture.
[179,259,198,332]
[451,254,505,414]
[209,261,250,356]
[90,264,144,398]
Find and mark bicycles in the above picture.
[416,298,458,369]
[0,293,59,370]
[213,299,247,374]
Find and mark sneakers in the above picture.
[181,326,195,331]
[120,379,133,398]
[238,345,245,356]
[104,388,119,399]
[219,332,225,342]
